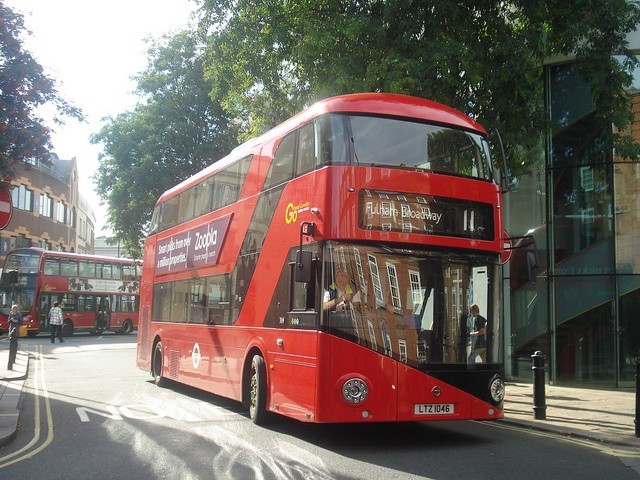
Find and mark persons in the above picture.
[7,304,22,341]
[323,267,364,312]
[468,304,487,364]
[351,273,385,309]
[47,300,65,343]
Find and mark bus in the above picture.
[136,92,541,425]
[0,246,143,337]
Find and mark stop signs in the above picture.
[0,186,13,231]
